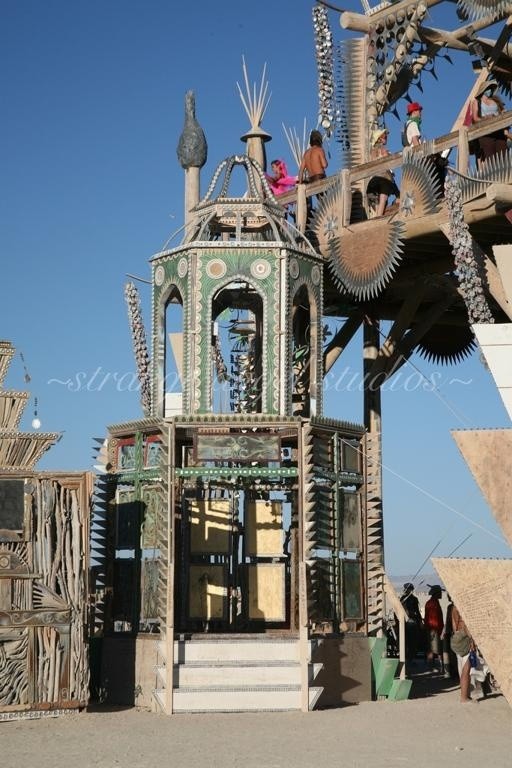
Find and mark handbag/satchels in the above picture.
[450,631,471,657]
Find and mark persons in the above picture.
[300,129,329,200]
[401,103,443,199]
[400,583,493,702]
[264,159,299,241]
[465,79,512,173]
[367,130,399,218]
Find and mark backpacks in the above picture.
[401,121,414,146]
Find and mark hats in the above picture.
[428,585,445,595]
[372,128,388,147]
[474,80,498,98]
[406,103,422,115]
